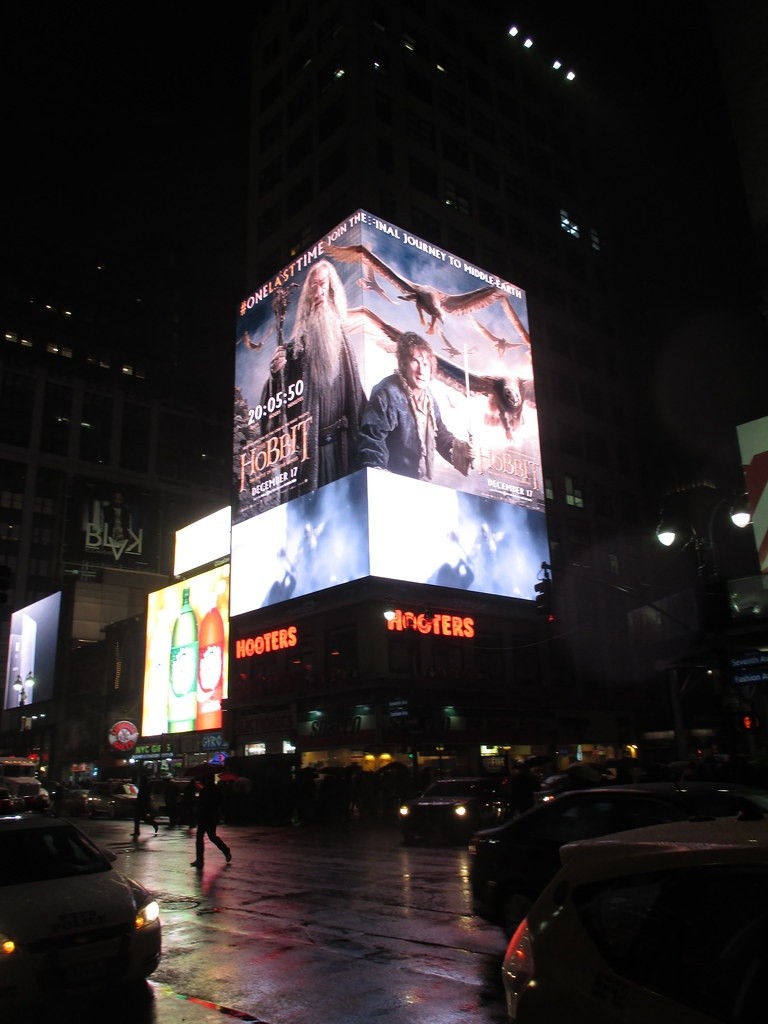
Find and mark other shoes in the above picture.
[190,860,203,865]
[153,823,158,832]
[224,848,232,862]
[131,833,140,836]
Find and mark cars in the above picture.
[468,778,768,944]
[0,812,163,1003]
[3,752,394,827]
[398,778,491,840]
[501,811,768,1024]
[497,749,611,807]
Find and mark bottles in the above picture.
[195,591,225,732]
[166,586,198,733]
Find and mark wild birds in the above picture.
[243,240,538,442]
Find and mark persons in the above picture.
[129,775,159,837]
[508,754,761,820]
[260,258,368,504]
[264,551,298,606]
[189,771,232,866]
[429,534,475,590]
[359,332,476,483]
